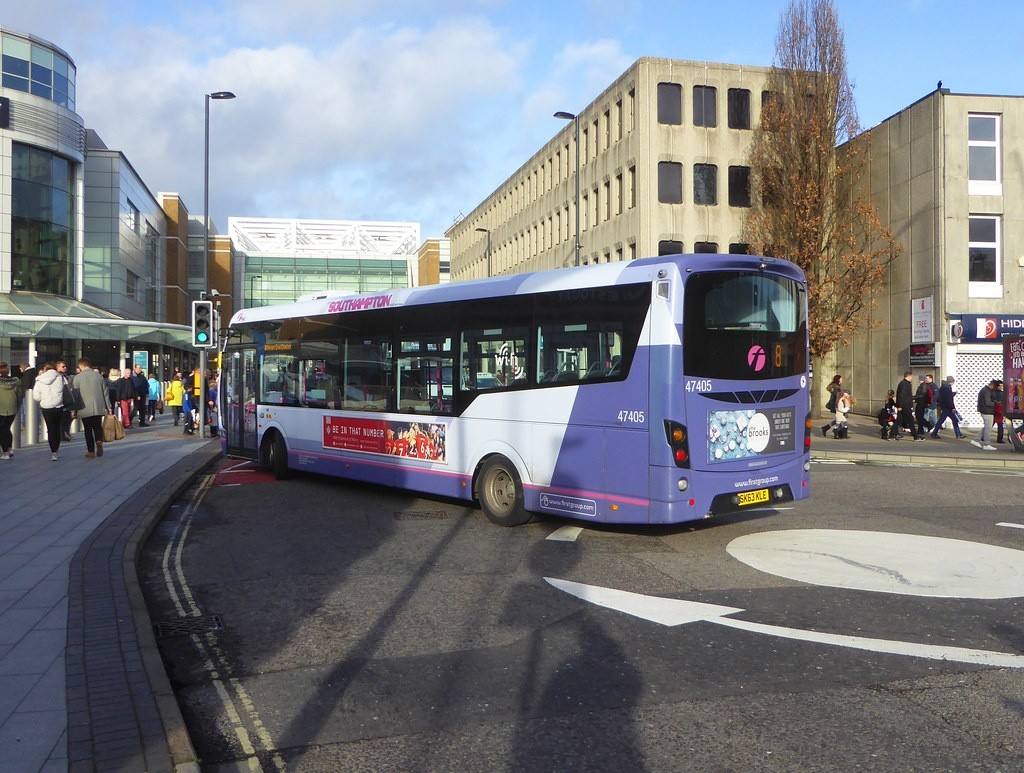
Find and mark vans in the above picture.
[419,365,467,402]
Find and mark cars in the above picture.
[466,372,502,389]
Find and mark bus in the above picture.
[215,253,812,527]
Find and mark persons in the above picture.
[969,379,997,450]
[822,375,848,437]
[101,367,115,415]
[75,367,81,374]
[878,371,926,442]
[911,373,934,434]
[117,368,141,429]
[981,380,1005,443]
[832,392,850,439]
[931,375,967,439]
[70,357,112,458]
[108,369,121,382]
[276,362,305,403]
[167,366,220,437]
[19,363,39,389]
[131,364,151,427]
[93,366,101,374]
[306,360,335,407]
[54,361,73,441]
[0,361,23,460]
[32,361,68,461]
[924,375,946,432]
[147,374,160,422]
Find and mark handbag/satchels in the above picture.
[156,391,218,429]
[102,414,125,443]
[825,401,830,409]
[953,409,963,422]
[61,375,75,408]
[923,408,937,427]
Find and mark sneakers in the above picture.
[970,440,983,448]
[983,444,998,451]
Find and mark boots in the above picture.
[839,427,843,439]
[821,424,831,437]
[842,426,849,439]
[833,429,840,439]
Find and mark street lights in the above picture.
[199,91,236,438]
[250,276,262,308]
[474,227,491,277]
[553,112,580,267]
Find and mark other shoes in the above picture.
[931,435,942,439]
[887,438,898,441]
[958,434,967,439]
[893,434,903,438]
[927,426,934,432]
[997,440,1005,443]
[917,431,925,434]
[914,436,925,441]
[0,412,221,460]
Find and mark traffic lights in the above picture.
[192,301,213,347]
